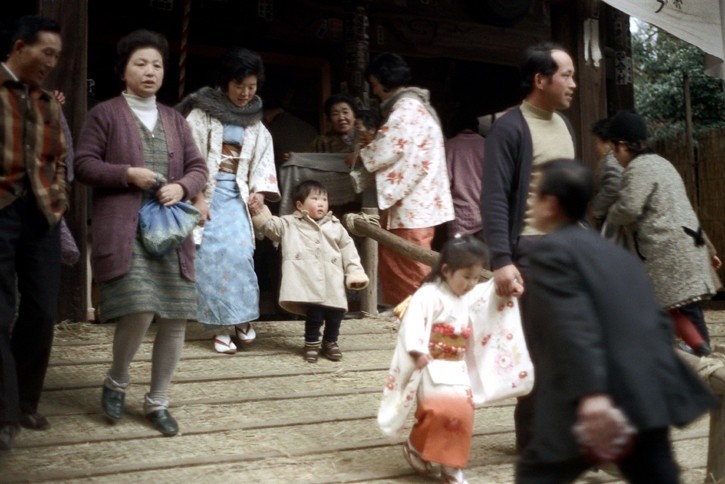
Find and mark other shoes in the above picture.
[693,342,711,357]
[304,345,319,362]
[102,372,128,421]
[321,339,342,359]
[144,393,178,434]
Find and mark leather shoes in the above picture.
[0,421,21,449]
[19,412,52,430]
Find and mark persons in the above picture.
[479,43,577,456]
[343,51,456,330]
[442,105,486,240]
[703,230,722,271]
[283,99,374,163]
[578,119,636,253]
[52,89,65,105]
[74,29,210,436]
[515,159,717,484]
[258,88,316,176]
[377,233,534,484]
[248,180,368,362]
[0,17,74,450]
[605,111,718,359]
[184,47,281,354]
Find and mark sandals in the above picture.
[212,335,237,354]
[235,322,255,345]
[439,464,469,484]
[403,440,433,476]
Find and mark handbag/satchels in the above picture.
[138,173,201,258]
[349,153,369,194]
[421,359,473,401]
